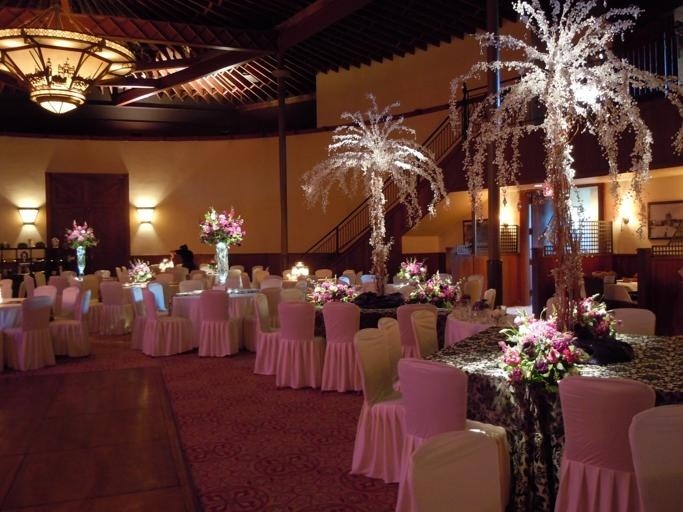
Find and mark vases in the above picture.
[74,244,87,276]
[212,239,231,286]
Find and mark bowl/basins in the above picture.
[0,242,11,249]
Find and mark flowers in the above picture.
[195,196,248,248]
[60,218,101,249]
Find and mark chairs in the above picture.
[0,258,276,371]
[254,268,495,392]
[353,272,682,511]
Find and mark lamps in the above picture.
[133,204,156,224]
[17,207,39,226]
[619,217,630,233]
[0,1,138,118]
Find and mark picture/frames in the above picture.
[645,200,682,241]
[461,217,488,249]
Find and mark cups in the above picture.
[452,299,506,325]
[52,239,58,248]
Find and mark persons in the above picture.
[168,243,196,273]
